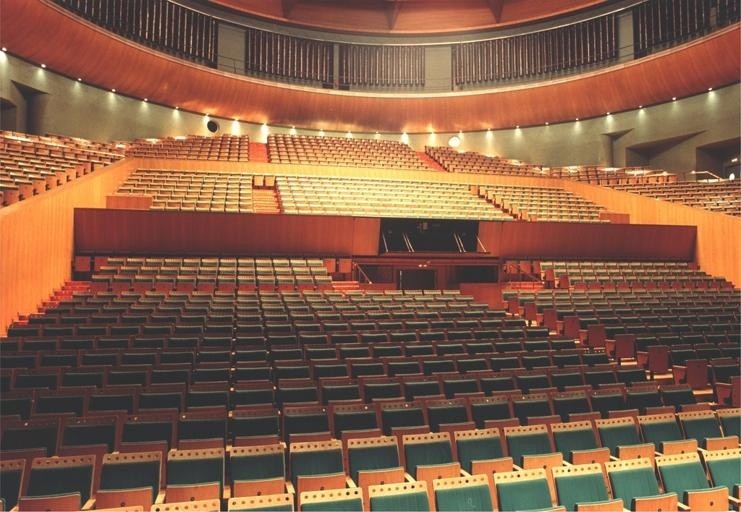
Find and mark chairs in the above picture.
[1,130,741,511]
